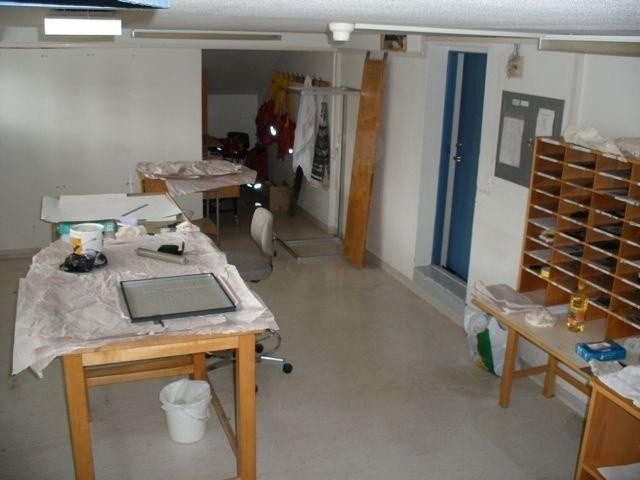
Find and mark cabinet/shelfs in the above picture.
[518,136,639,342]
[575,370,640,479]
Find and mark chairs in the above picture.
[204,206,293,395]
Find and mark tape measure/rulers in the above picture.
[122,203,148,218]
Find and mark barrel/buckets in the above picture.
[69,223,105,258]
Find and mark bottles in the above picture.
[566,280,589,333]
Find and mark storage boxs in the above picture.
[265,180,290,213]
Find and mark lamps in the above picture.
[44,8,123,36]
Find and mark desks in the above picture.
[135,160,258,246]
[49,192,275,477]
[471,287,639,409]
